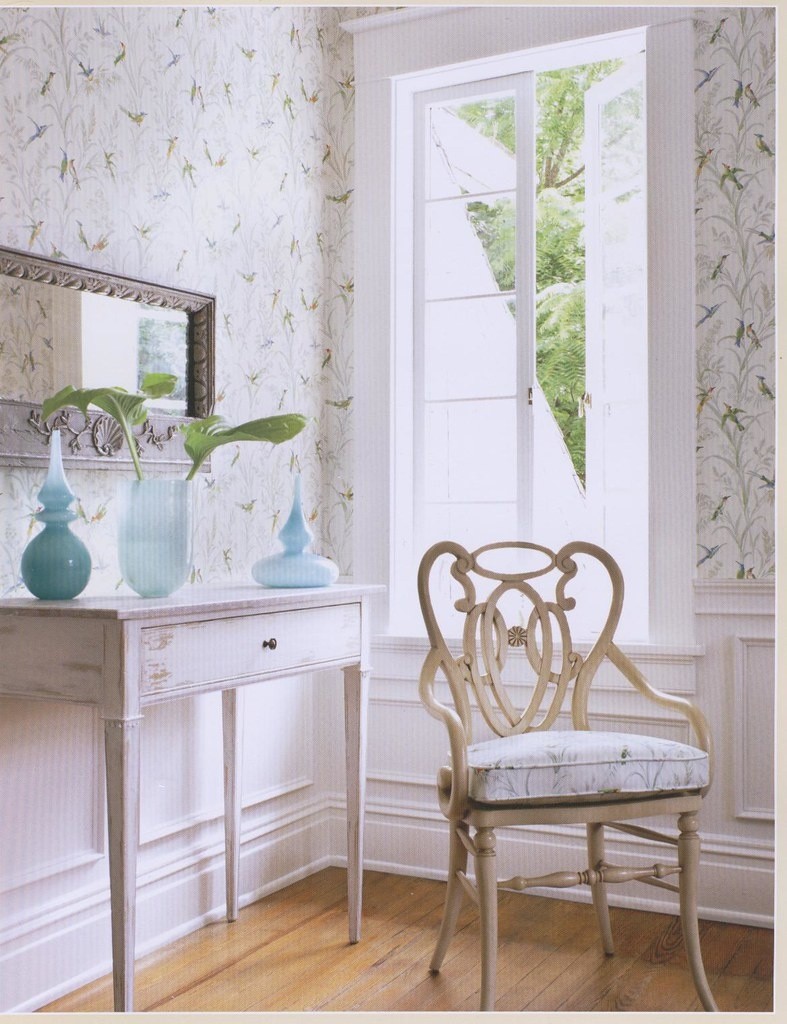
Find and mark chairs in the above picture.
[415,539,720,1013]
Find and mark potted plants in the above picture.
[40,370,305,599]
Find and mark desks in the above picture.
[0,579,375,1011]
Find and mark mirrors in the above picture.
[2,244,218,470]
